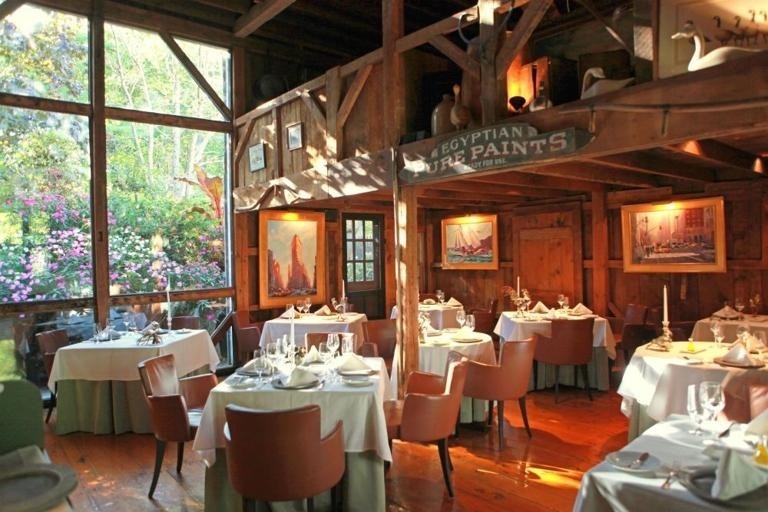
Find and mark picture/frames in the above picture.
[258,210,327,310]
[247,140,266,173]
[621,196,728,274]
[441,215,499,271]
[657,0,768,79]
[286,121,304,152]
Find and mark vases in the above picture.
[430,93,454,138]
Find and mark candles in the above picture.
[662,285,667,319]
[342,279,345,297]
[517,275,520,295]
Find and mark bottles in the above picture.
[686,338,695,353]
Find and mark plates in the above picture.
[607,450,663,473]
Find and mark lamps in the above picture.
[750,157,764,173]
[674,140,701,157]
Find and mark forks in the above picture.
[660,460,682,488]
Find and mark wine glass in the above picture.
[425,311,482,346]
[236,332,378,392]
[514,294,597,321]
[423,288,445,305]
[287,296,347,321]
[686,381,727,439]
[716,294,767,325]
[94,312,145,343]
[707,324,768,353]
